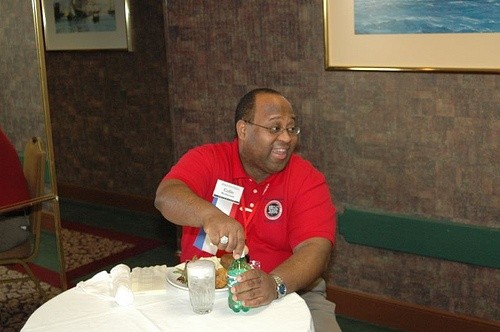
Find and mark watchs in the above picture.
[271,274,286,299]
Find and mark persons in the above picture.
[0,128,31,252]
[154,88,341,332]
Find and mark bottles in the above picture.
[226,249,251,313]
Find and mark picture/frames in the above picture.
[323,1,500,74]
[41,0,134,53]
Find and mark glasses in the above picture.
[244,120,301,138]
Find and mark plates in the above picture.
[166,263,229,291]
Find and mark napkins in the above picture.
[76,270,115,301]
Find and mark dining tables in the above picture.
[17,265,316,332]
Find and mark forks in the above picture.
[176,261,190,283]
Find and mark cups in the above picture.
[250,260,261,269]
[187,260,215,315]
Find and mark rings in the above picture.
[220,236,228,244]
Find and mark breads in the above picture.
[215,254,235,289]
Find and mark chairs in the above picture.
[0,136,55,298]
